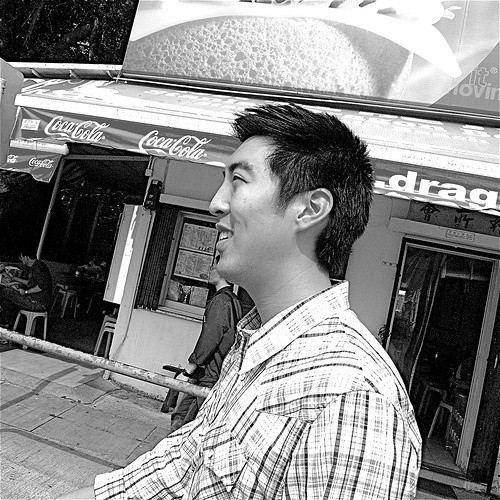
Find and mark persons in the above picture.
[2,250,54,344]
[54,104,423,500]
[170,254,242,432]
[88,254,106,275]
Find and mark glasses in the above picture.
[210,265,216,271]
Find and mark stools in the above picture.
[9,310,47,350]
[94,314,117,357]
[427,400,454,449]
[50,289,77,318]
[418,383,447,424]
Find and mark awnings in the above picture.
[0,79,500,214]
